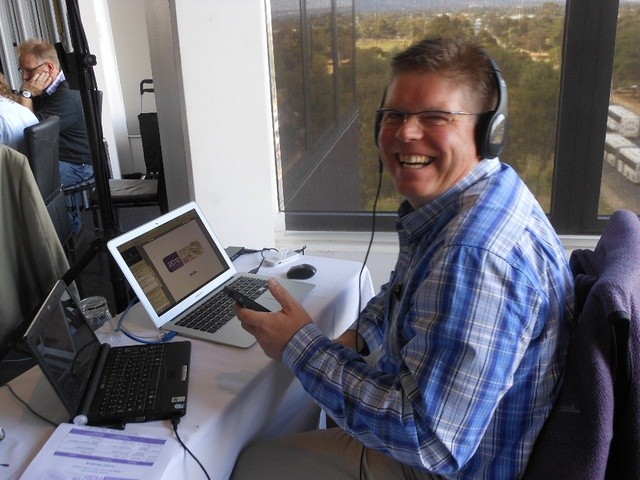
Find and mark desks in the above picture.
[1,244,376,480]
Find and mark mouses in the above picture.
[286,263,316,282]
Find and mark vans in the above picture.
[606,105,640,139]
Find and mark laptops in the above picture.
[105,199,317,351]
[20,276,193,433]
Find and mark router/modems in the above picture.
[264,249,300,266]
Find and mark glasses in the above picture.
[375,109,481,126]
[18,62,48,76]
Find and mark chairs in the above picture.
[61,90,121,253]
[23,117,87,299]
[528,209,640,479]
[90,128,175,233]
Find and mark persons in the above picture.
[233,35,576,478]
[17,40,96,244]
[0,94,39,157]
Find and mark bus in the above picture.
[604,133,640,186]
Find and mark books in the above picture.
[19,423,182,480]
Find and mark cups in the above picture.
[78,296,119,349]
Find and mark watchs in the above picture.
[23,89,39,100]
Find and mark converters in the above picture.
[222,246,244,261]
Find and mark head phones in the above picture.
[361,53,523,158]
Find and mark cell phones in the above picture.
[221,283,270,314]
